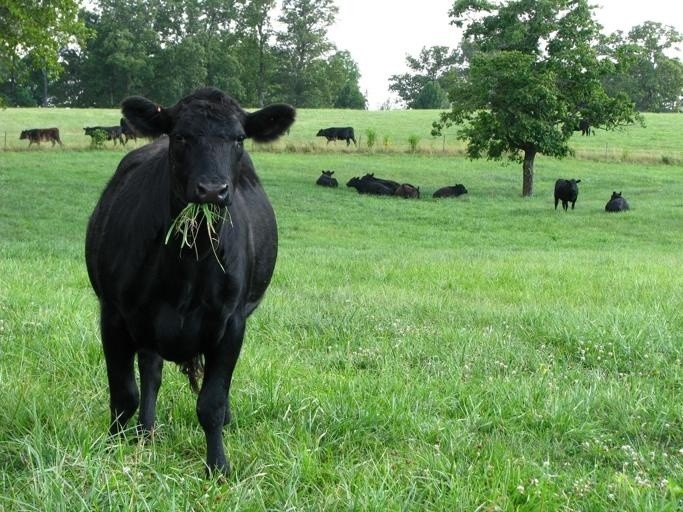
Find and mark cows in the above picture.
[396,182,420,199]
[553,176,582,212]
[121,116,152,143]
[316,126,357,147]
[604,189,630,212]
[316,170,339,187]
[432,183,467,198]
[346,176,391,194]
[361,173,401,192]
[84,87,296,485]
[83,125,124,147]
[19,127,62,148]
